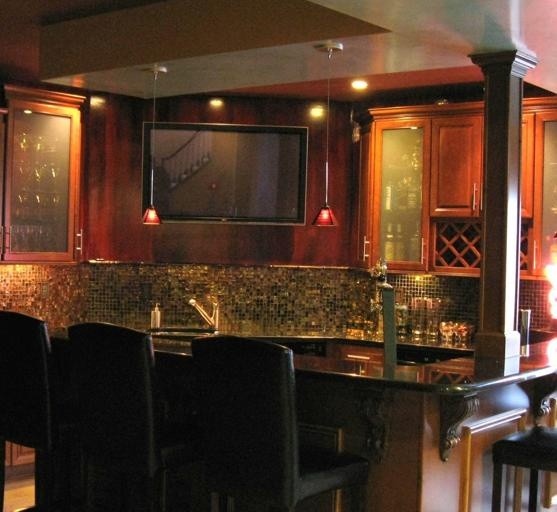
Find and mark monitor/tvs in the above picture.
[142,120,309,227]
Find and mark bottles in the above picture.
[381,168,420,211]
[383,221,421,263]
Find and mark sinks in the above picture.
[149,332,210,343]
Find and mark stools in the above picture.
[490,422,557,511]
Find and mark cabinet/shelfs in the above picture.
[427,109,534,218]
[0,78,88,267]
[347,104,430,273]
[533,104,556,285]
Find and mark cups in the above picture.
[410,295,441,340]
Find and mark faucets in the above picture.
[188,298,221,330]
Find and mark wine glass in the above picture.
[439,321,469,350]
[12,131,62,253]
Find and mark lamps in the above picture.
[310,39,342,230]
[136,61,164,226]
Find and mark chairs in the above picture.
[63,318,200,512]
[190,330,371,511]
[0,307,61,511]
